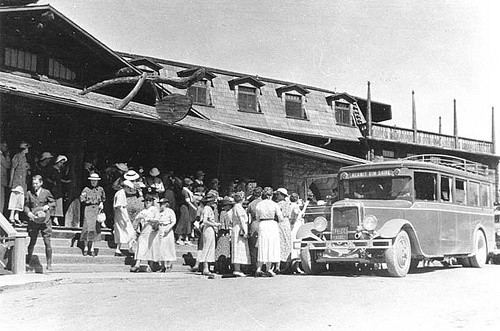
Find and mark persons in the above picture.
[23,175,57,272]
[0,138,73,227]
[78,151,340,279]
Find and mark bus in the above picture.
[292,153,495,277]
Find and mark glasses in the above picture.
[146,199,151,202]
[277,192,281,196]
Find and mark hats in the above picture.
[196,170,205,177]
[194,187,206,195]
[40,152,53,161]
[142,194,156,202]
[202,193,218,201]
[150,168,160,177]
[54,154,67,163]
[115,162,128,171]
[212,179,220,182]
[277,188,288,196]
[123,180,133,189]
[157,198,169,204]
[88,174,101,181]
[32,207,51,224]
[124,170,140,180]
[183,178,194,185]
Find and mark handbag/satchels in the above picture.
[96,209,106,223]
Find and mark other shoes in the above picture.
[46,265,53,271]
[83,251,88,256]
[130,265,171,272]
[89,250,96,256]
[115,251,124,256]
[231,268,277,277]
